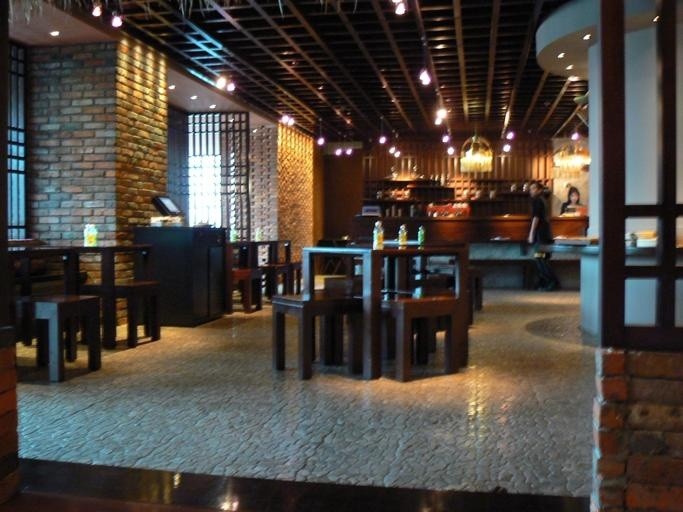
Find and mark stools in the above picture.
[231,267,265,314]
[319,275,384,366]
[272,293,362,375]
[380,286,465,382]
[262,261,303,300]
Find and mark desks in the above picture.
[8,244,150,350]
[302,245,469,380]
[234,239,292,310]
[134,226,231,327]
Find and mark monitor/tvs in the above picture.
[152,196,184,216]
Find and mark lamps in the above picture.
[92,0,126,29]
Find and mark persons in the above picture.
[559,186,589,237]
[526,181,561,292]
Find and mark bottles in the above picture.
[83,224,96,247]
[425,203,471,218]
[375,190,410,200]
[624,233,636,248]
[461,189,496,201]
[384,204,414,218]
[417,225,426,249]
[521,182,529,192]
[372,220,384,250]
[510,183,517,192]
[397,223,407,248]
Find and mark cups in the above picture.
[255,228,265,242]
[231,230,237,241]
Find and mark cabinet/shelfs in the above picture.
[361,177,551,216]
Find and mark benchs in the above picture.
[80,278,163,348]
[10,295,102,382]
